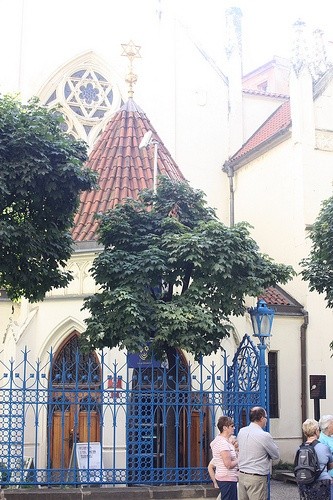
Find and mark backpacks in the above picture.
[293,440,326,484]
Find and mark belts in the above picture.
[239,471,268,476]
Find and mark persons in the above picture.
[236,407,279,499]
[317,415,333,498]
[208,416,239,500]
[294,419,333,500]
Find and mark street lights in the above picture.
[248,299,275,432]
